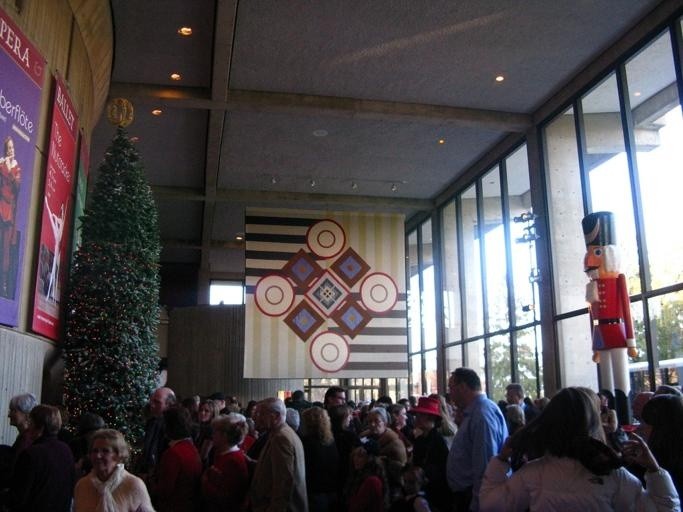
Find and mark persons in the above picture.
[0,136,20,298]
[45,196,69,307]
[582,211,638,443]
[1,369,683,512]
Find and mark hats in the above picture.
[407,397,446,423]
